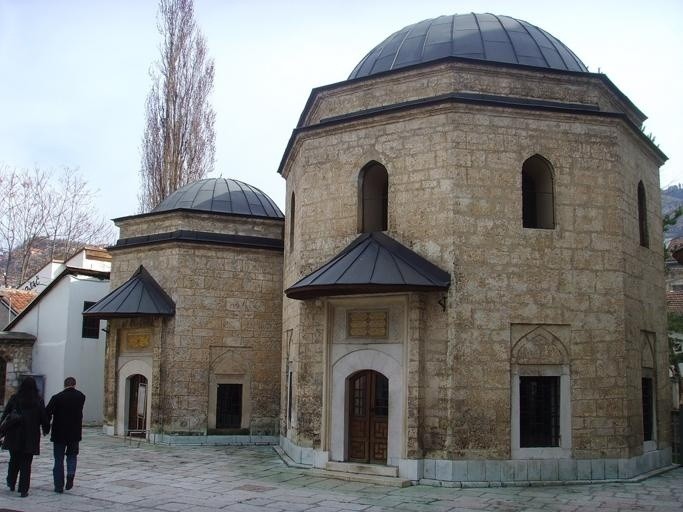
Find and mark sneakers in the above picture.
[10,486,15,491]
[54,486,72,492]
[21,492,29,497]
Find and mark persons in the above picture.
[0,375,86,498]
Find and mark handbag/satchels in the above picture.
[4,409,23,439]
